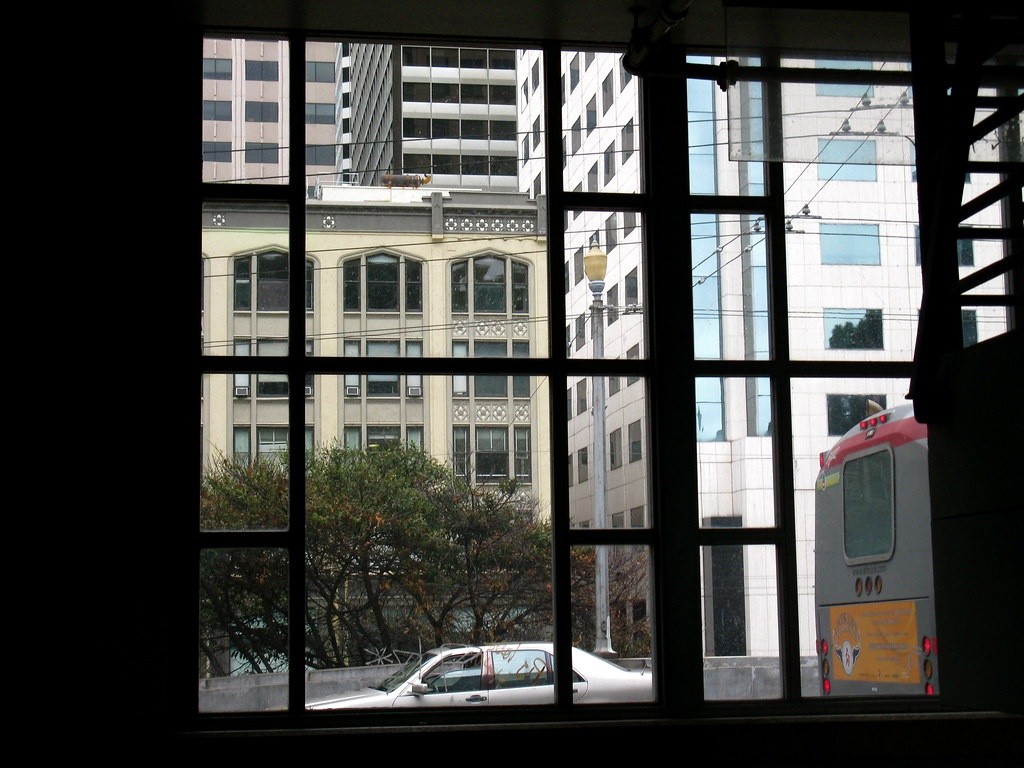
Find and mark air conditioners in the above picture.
[305,387,311,395]
[407,388,420,397]
[347,386,358,396]
[236,388,248,396]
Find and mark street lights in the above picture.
[582,235,616,657]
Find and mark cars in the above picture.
[308,642,654,706]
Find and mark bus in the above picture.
[814,403,941,695]
